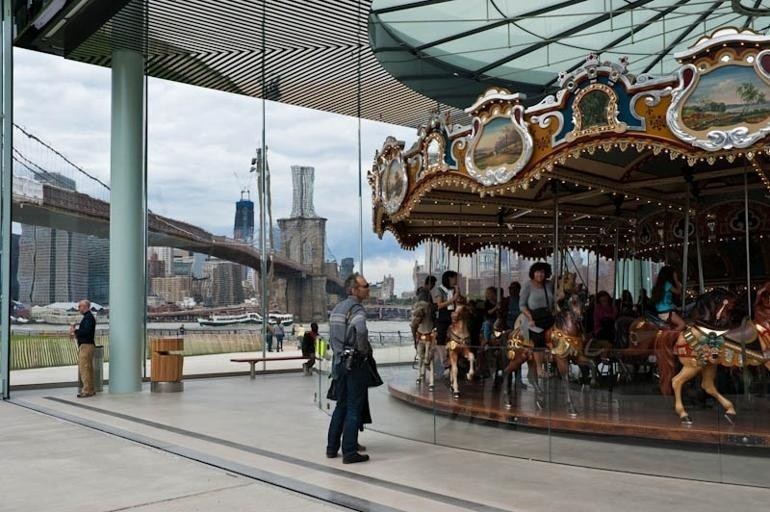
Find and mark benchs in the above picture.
[230,354,327,379]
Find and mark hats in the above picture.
[529,262,552,280]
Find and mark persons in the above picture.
[72,299,97,396]
[300,323,319,376]
[265,321,273,352]
[327,271,371,463]
[588,266,685,339]
[274,321,286,352]
[292,325,296,336]
[416,262,553,379]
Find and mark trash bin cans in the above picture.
[77,345,104,392]
[148,337,184,393]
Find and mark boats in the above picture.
[197,312,294,327]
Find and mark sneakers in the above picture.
[327,443,369,464]
[77,393,93,397]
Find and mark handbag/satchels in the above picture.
[334,348,367,378]
[532,308,552,324]
